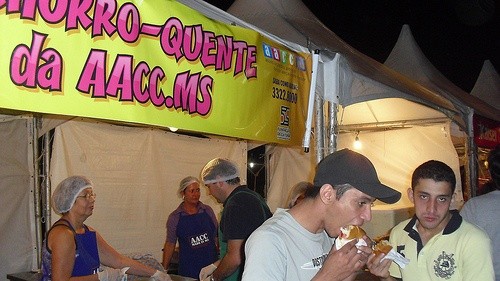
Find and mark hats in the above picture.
[312,147,402,204]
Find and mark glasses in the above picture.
[76,192,96,201]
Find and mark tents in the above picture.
[227,0,500,244]
[0,0,319,281]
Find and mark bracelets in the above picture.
[210,275,214,281]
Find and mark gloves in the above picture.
[150,269,172,281]
[98,266,124,280]
[198,263,218,281]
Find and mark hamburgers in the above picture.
[374,239,393,256]
[339,225,366,239]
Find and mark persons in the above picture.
[162,148,500,281]
[38,176,172,281]
